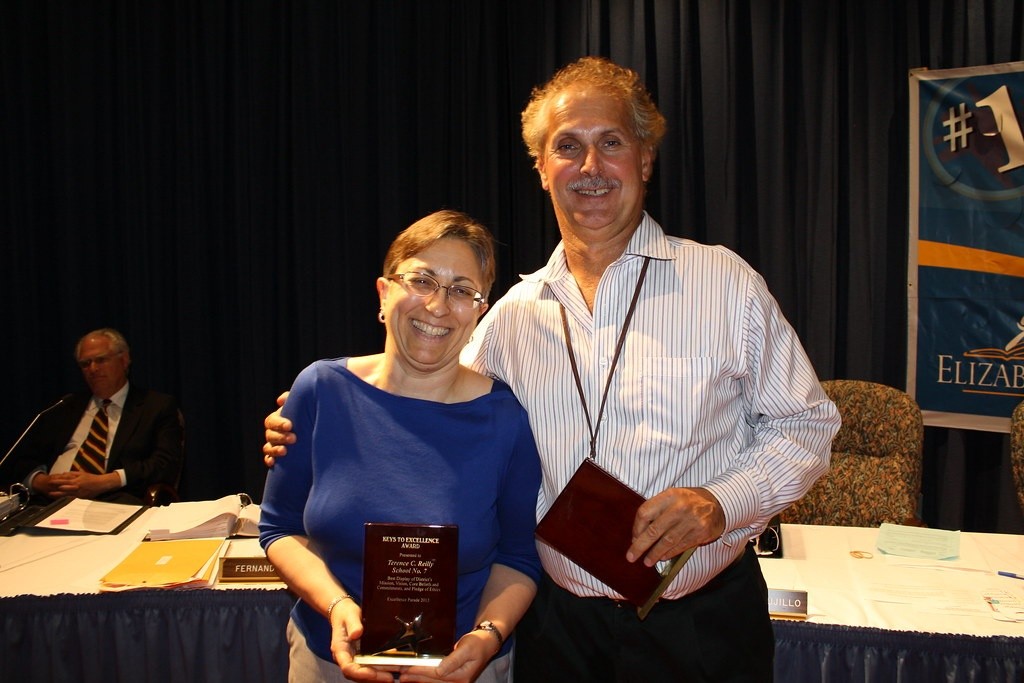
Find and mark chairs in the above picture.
[779,380,926,526]
[142,392,191,504]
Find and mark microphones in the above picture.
[0,393,76,466]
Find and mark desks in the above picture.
[0,492,1024,683]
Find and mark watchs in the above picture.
[471,619,502,656]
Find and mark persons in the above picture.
[263,55,841,682]
[23,327,177,509]
[260,209,543,683]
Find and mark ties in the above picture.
[70,398,111,475]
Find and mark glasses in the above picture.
[387,271,488,309]
[77,351,122,369]
